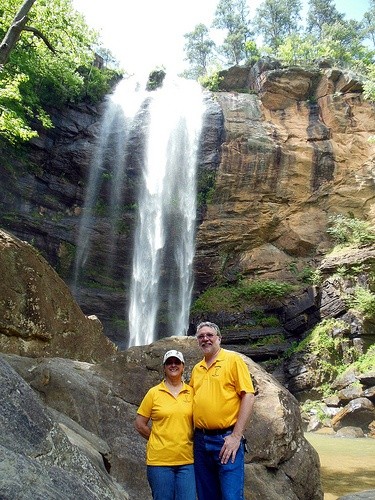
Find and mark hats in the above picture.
[163,349,186,365]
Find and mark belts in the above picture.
[194,426,237,436]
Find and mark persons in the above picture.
[189,322,255,500]
[134,349,196,500]
